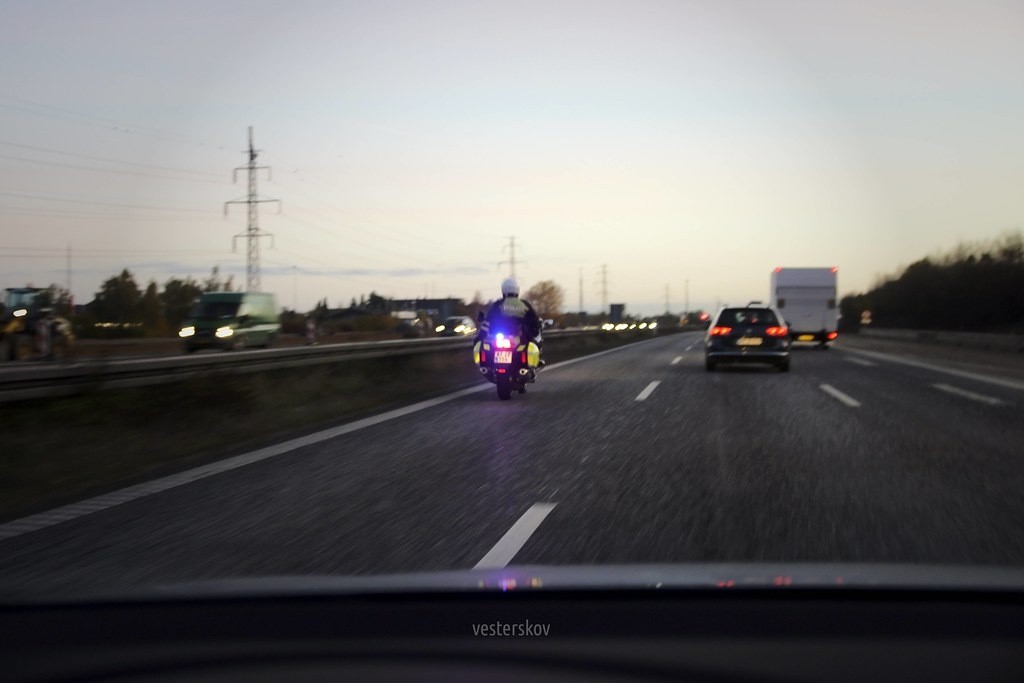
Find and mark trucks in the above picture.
[769,266,840,346]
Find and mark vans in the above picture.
[180,291,283,353]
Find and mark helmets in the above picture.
[502,279,520,294]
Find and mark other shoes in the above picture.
[538,360,545,367]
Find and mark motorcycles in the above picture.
[473,309,555,402]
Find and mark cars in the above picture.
[436,315,476,338]
[704,306,791,371]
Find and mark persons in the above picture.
[474,278,545,382]
